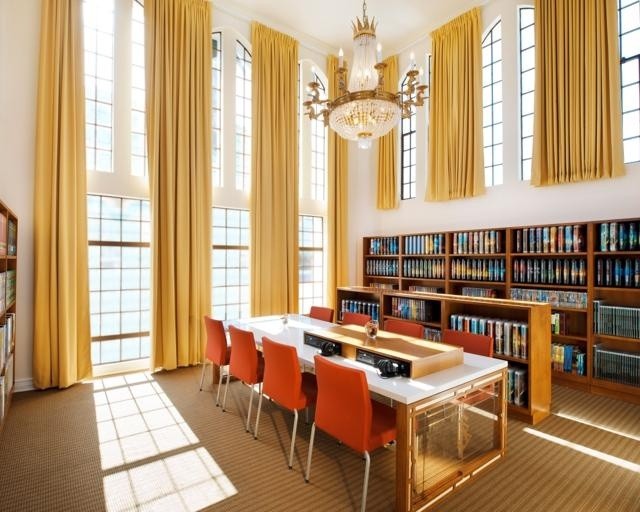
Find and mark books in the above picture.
[1,213,17,421]
[342,221,640,414]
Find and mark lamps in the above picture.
[301,0,430,150]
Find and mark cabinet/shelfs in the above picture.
[0,197,19,435]
[337,286,552,426]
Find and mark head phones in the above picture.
[321,341,338,356]
[378,359,399,376]
